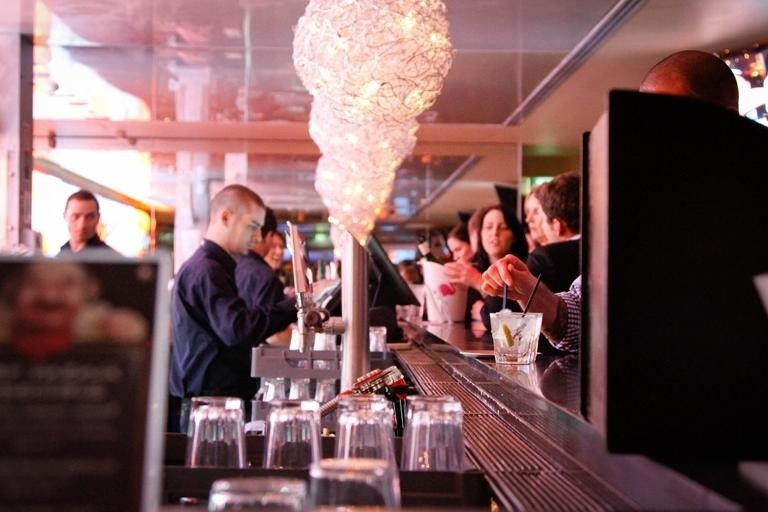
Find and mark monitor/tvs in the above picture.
[367,231,421,307]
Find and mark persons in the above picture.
[56,191,122,258]
[234,206,292,348]
[480,50,738,351]
[264,230,286,270]
[444,204,529,320]
[396,207,481,286]
[524,183,547,247]
[479,171,579,330]
[168,183,298,435]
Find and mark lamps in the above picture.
[312,155,395,261]
[306,97,420,180]
[291,1,453,125]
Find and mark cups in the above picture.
[333,395,397,459]
[403,397,470,468]
[265,400,321,470]
[186,397,243,469]
[488,311,540,365]
[309,460,402,511]
[207,477,304,511]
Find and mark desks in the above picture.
[167,326,639,512]
[422,319,768,511]
